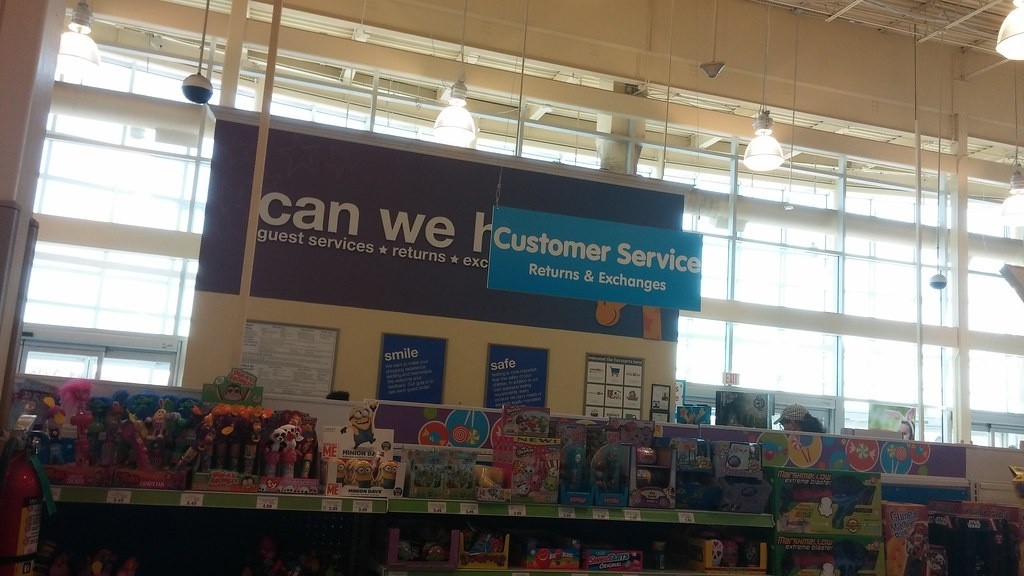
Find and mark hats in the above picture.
[774,404,809,425]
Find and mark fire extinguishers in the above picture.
[0,429,53,576]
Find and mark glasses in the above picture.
[779,421,790,430]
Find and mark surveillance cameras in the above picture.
[181,74,213,104]
[930,275,947,290]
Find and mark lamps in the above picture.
[995,0,1024,61]
[434,0,476,138]
[743,0,785,171]
[60,0,100,67]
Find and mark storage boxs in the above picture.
[492,405,775,576]
[768,531,884,576]
[715,391,772,429]
[882,499,1024,576]
[768,466,882,534]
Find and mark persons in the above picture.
[774,404,826,433]
[900,421,914,440]
